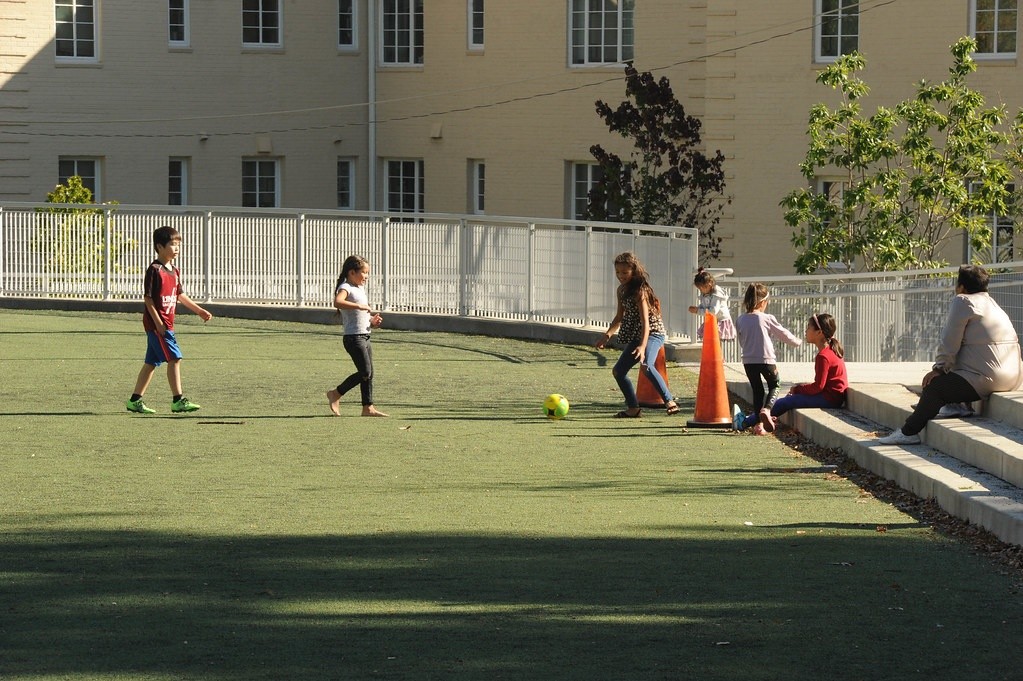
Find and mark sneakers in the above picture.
[171,395,200,413]
[755,423,767,435]
[733,404,746,430]
[936,403,973,417]
[759,408,777,432]
[127,398,156,415]
[879,428,921,445]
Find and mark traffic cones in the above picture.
[686,308,733,428]
[636,344,670,408]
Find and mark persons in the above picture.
[326,254,389,418]
[735,282,803,435]
[878,265,1023,445]
[127,226,212,414]
[688,266,736,341]
[596,253,680,417]
[732,314,848,431]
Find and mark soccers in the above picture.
[543,394,569,419]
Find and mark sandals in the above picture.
[613,408,641,418]
[667,398,681,415]
